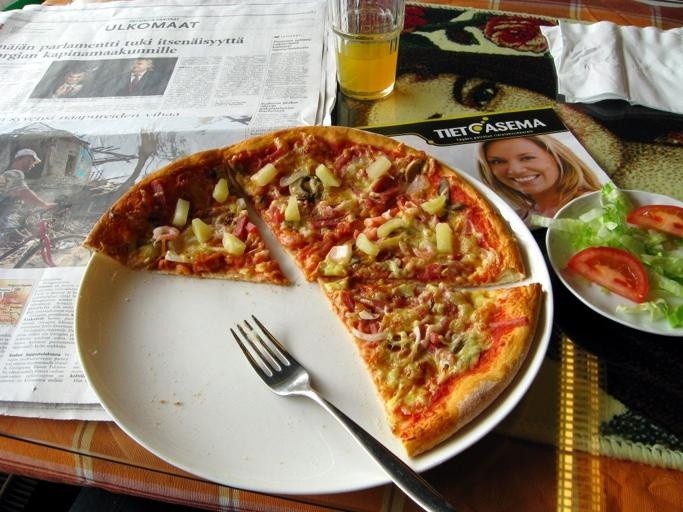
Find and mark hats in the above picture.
[15,148,41,165]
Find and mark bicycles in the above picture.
[0,204,93,268]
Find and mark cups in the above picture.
[324,0,406,101]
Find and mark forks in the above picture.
[228,316,454,508]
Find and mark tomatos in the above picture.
[628,204,683,238]
[568,244,651,304]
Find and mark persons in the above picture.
[477,134,603,231]
[0,148,58,250]
[39,68,90,98]
[332,28,682,203]
[103,57,158,96]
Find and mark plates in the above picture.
[69,141,555,497]
[546,188,683,336]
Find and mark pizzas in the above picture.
[81,125,542,458]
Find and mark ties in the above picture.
[131,73,140,89]
